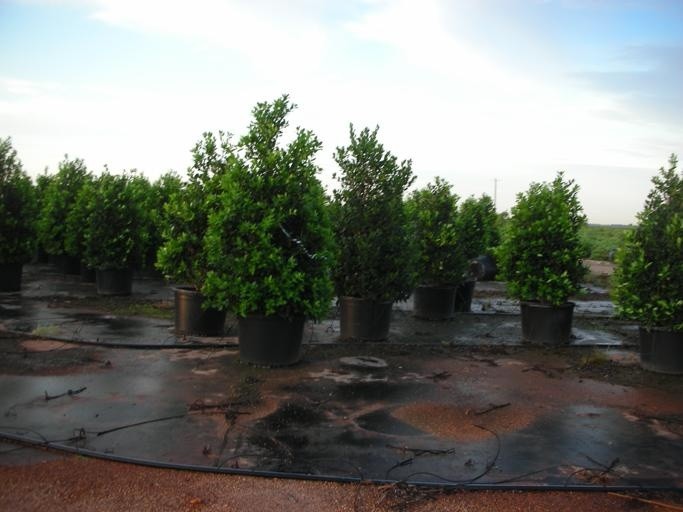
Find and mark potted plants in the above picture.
[83,174,148,294]
[0,143,37,293]
[444,216,477,312]
[407,177,466,321]
[607,156,683,374]
[315,123,415,340]
[202,93,337,368]
[155,131,250,336]
[496,171,590,343]
